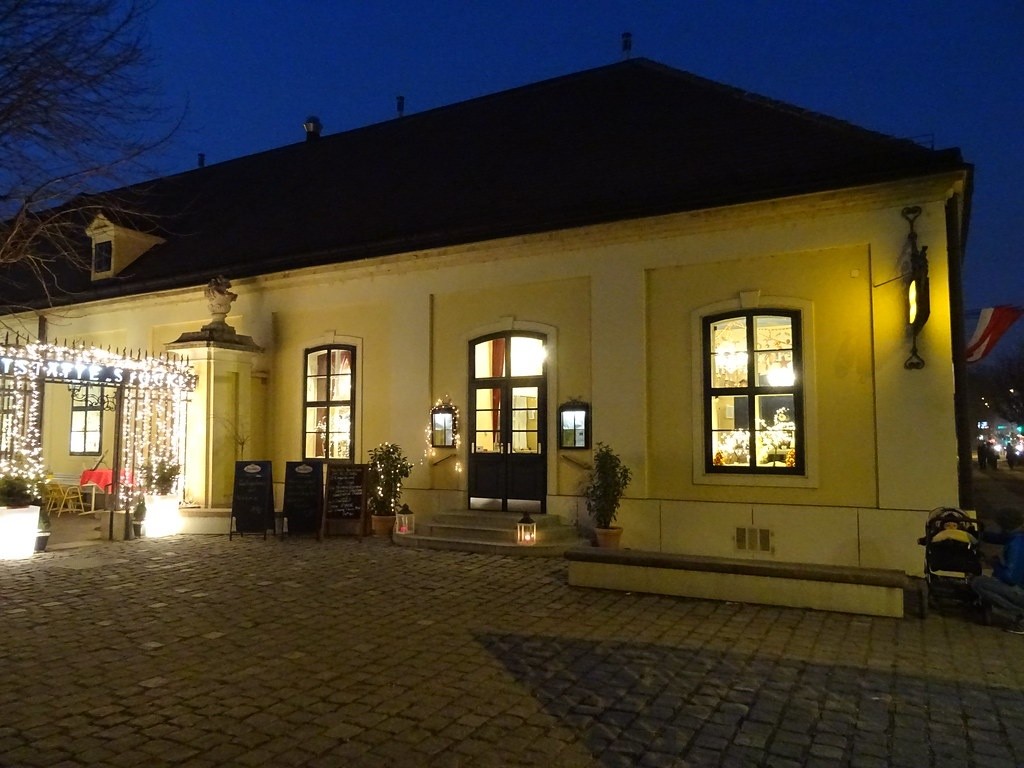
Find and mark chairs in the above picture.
[46,481,85,517]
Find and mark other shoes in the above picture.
[1004,623,1024,635]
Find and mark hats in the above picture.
[940,513,958,527]
[993,508,1023,529]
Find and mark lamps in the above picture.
[396,503,415,535]
[516,512,537,546]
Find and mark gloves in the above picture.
[983,555,999,566]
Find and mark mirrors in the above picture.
[557,398,592,450]
[428,403,456,449]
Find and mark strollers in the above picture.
[916,506,984,617]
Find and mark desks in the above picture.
[78,469,138,516]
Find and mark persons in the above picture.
[1006,443,1015,468]
[977,442,999,472]
[940,514,959,531]
[971,508,1024,634]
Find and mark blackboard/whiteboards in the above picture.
[324,464,368,520]
[284,462,322,520]
[232,461,271,519]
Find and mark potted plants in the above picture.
[561,440,633,548]
[132,492,146,539]
[29,470,54,553]
[366,441,413,538]
